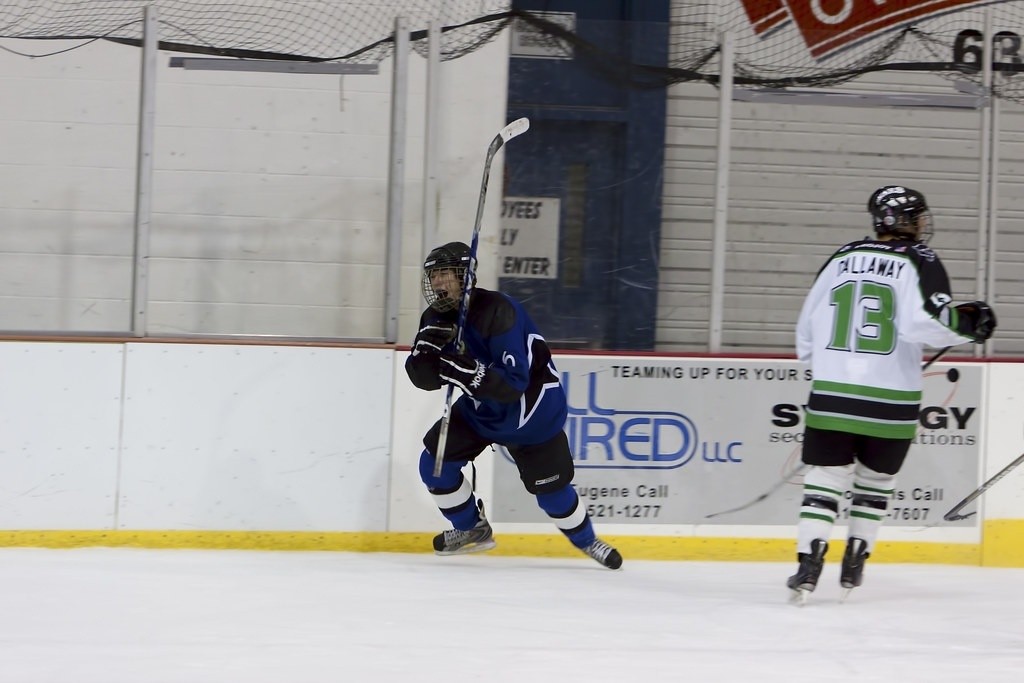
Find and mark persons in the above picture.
[787,186,997,605]
[405,242,623,571]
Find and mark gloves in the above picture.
[972,299,997,344]
[409,320,456,360]
[417,338,478,394]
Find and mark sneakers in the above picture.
[834,536,872,600]
[430,500,498,554]
[781,537,826,604]
[579,535,623,570]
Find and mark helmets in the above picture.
[868,185,934,247]
[421,236,476,313]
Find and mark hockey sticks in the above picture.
[943,453,1024,522]
[921,345,954,371]
[432,116,532,478]
